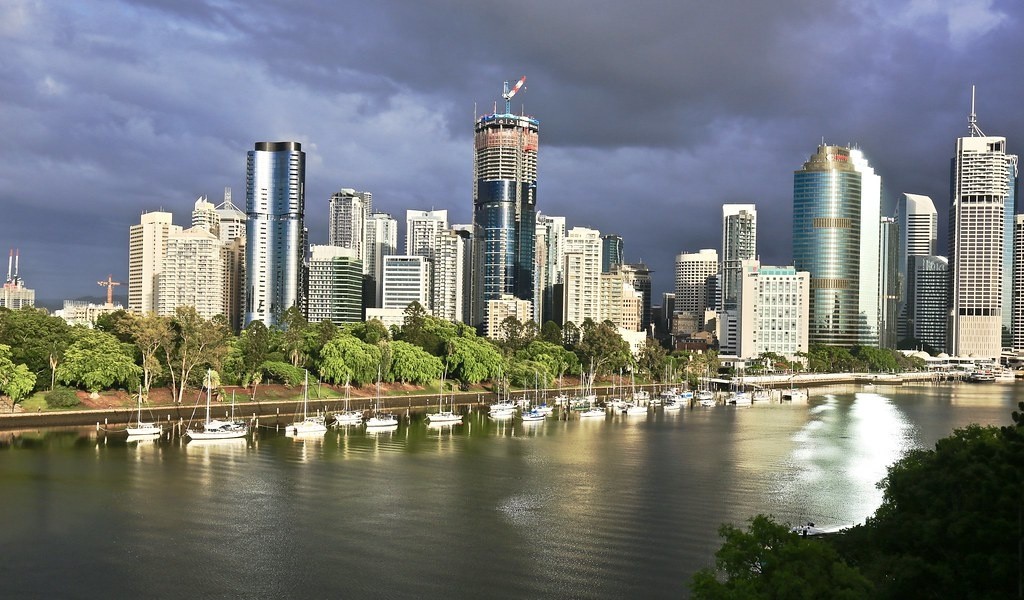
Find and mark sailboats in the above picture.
[489,356,807,420]
[366,364,398,427]
[285,370,328,433]
[333,373,364,421]
[186,367,248,440]
[126,383,161,435]
[426,372,464,422]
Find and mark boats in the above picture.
[126,434,160,446]
